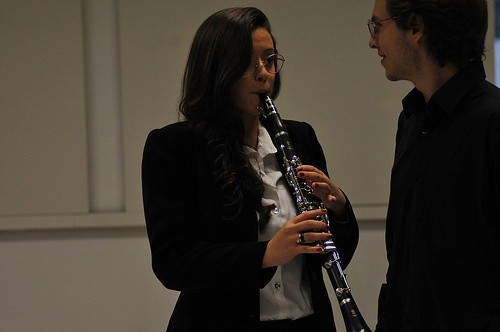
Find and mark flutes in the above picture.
[257,94,370,332]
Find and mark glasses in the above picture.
[241,54,285,79]
[367,11,414,39]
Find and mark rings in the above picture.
[296,234,306,245]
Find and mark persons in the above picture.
[138,4,360,331]
[365,0,500,331]
